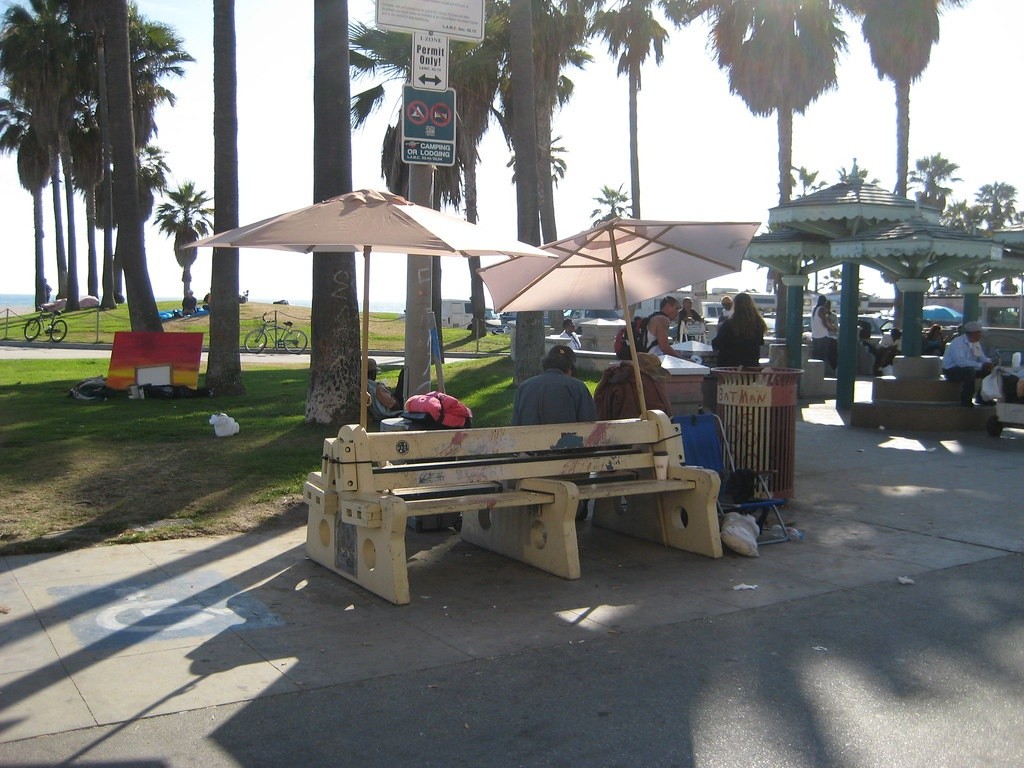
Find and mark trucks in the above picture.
[440,299,501,331]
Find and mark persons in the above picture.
[45,279,52,303]
[560,319,582,350]
[875,329,902,374]
[942,321,997,408]
[920,324,962,356]
[367,358,404,422]
[594,327,674,424]
[512,345,598,426]
[614,293,767,367]
[60,266,68,298]
[114,288,124,303]
[182,288,211,315]
[811,295,838,378]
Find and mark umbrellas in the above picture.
[179,189,560,434]
[476,218,761,550]
[922,305,963,320]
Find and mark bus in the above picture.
[747,293,778,316]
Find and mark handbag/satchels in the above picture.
[980,374,1002,401]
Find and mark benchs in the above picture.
[302,409,723,607]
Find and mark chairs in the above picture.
[670,413,788,546]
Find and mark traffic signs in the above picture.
[411,32,449,92]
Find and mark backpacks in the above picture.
[614,312,669,360]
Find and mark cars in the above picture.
[765,314,896,347]
[563,307,618,335]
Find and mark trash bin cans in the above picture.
[710,367,804,503]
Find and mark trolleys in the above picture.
[985,348,1024,437]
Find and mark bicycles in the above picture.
[244,312,308,355]
[24,307,67,343]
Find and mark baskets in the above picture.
[254,320,265,331]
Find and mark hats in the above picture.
[368,358,382,371]
[964,321,988,332]
[548,345,577,376]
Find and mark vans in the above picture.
[700,302,724,319]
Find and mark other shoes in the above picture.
[961,397,974,407]
[975,398,996,405]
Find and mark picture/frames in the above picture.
[134,362,174,386]
